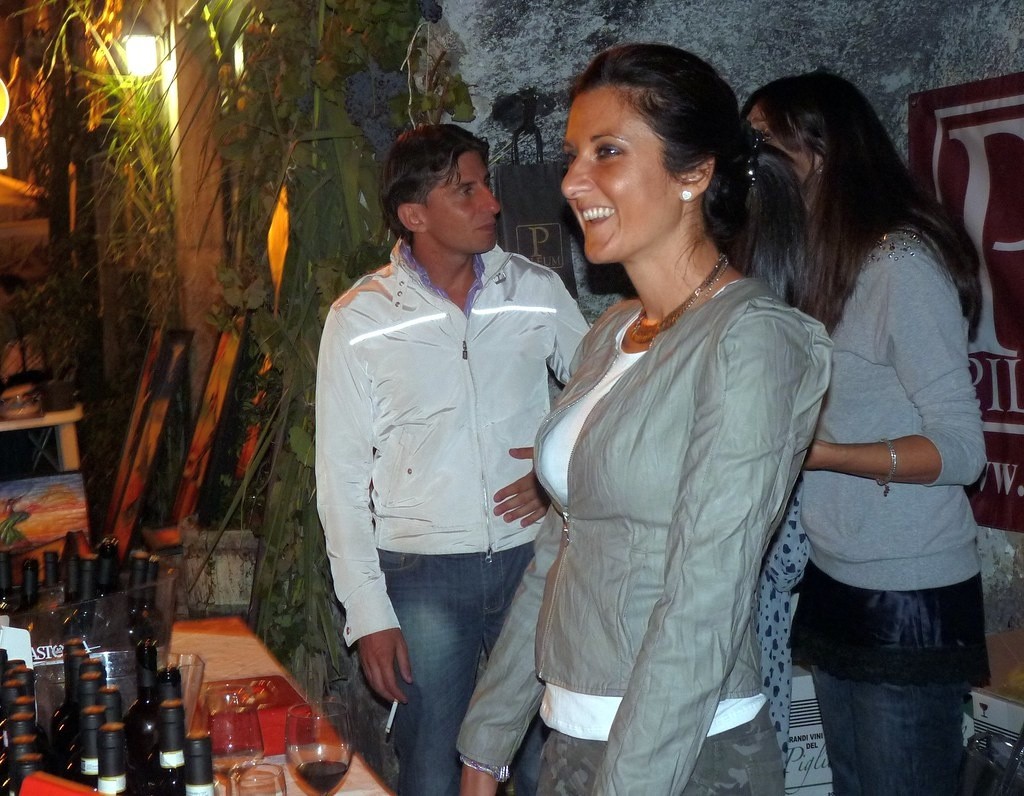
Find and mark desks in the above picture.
[167,618,395,796]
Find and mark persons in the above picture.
[737,68,990,795]
[319,123,597,796]
[451,41,834,796]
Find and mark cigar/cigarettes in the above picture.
[381,699,403,743]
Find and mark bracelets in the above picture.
[875,438,898,487]
[459,754,512,782]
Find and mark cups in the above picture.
[284,701,354,796]
[228,763,288,796]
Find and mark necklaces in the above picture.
[629,253,730,343]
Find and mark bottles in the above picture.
[0,543,214,796]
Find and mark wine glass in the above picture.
[202,684,264,796]
